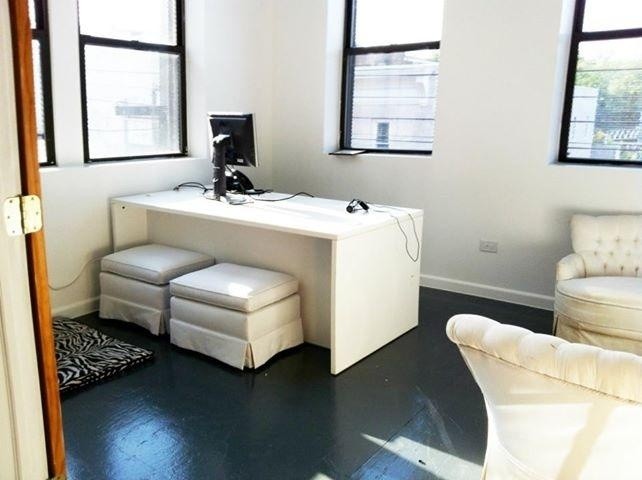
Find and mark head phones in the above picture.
[346,198,369,213]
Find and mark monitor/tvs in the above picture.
[206,112,257,201]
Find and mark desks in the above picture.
[109,184,423,376]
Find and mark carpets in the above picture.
[52,316,156,399]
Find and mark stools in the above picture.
[168,262,304,371]
[97,244,215,336]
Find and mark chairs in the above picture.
[444,313,642,480]
[553,214,642,355]
